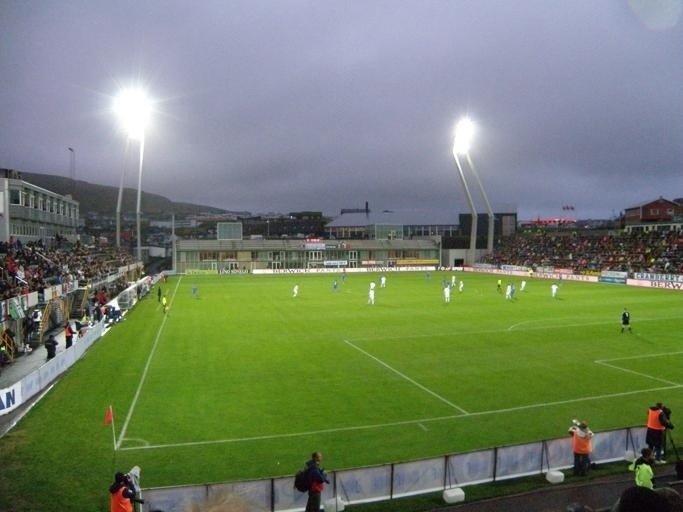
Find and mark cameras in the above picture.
[572,418,581,428]
[132,494,145,505]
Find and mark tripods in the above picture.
[653,419,681,462]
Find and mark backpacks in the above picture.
[295,468,317,492]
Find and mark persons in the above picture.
[107,469,144,512]
[474,226,681,278]
[439,274,464,303]
[644,402,675,464]
[634,449,657,488]
[496,279,526,299]
[365,281,376,306]
[0,225,168,378]
[426,271,430,280]
[291,283,300,299]
[379,276,386,288]
[291,451,330,512]
[375,273,381,286]
[551,282,558,297]
[330,279,338,293]
[619,307,633,334]
[341,273,346,282]
[566,460,682,512]
[570,420,592,474]
[190,285,198,298]
